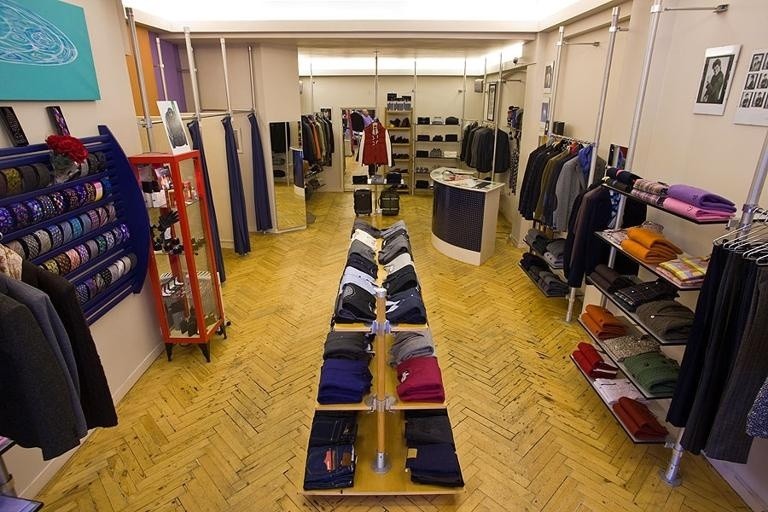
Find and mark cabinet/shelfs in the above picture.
[127,150,226,363]
[384,107,458,192]
[298,219,466,497]
[1,126,151,328]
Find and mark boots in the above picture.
[389,117,401,129]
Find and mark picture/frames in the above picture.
[487,84,496,121]
[692,44,768,126]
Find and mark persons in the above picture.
[361,108,373,127]
[740,54,768,108]
[702,59,724,103]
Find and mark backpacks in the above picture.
[378,189,401,217]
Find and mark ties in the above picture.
[0,151,139,304]
[0,108,28,144]
[51,107,71,136]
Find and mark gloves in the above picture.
[156,210,179,231]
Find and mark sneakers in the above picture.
[392,151,411,160]
[391,167,409,175]
[401,117,411,128]
[391,134,409,144]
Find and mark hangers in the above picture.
[711,206,768,265]
[546,133,594,153]
[480,120,496,130]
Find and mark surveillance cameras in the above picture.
[513,57,519,64]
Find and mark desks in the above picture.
[429,166,505,266]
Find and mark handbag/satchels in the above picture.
[387,171,403,185]
[372,173,385,186]
[415,177,430,190]
[432,116,444,126]
[445,116,459,126]
[443,150,459,160]
[417,134,431,142]
[416,116,431,126]
[445,133,458,142]
[415,164,429,175]
[416,149,429,158]
[429,147,442,159]
[351,173,369,185]
[432,135,444,142]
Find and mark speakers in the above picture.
[475,79,483,92]
[353,175,368,184]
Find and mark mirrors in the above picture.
[270,121,306,232]
[341,107,375,184]
